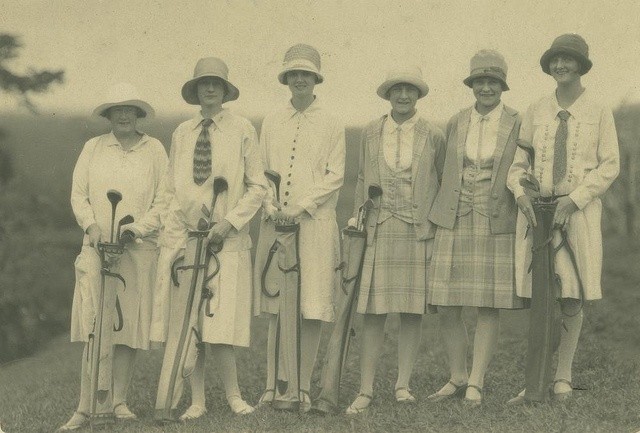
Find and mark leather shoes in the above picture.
[462,384,484,408]
[505,386,525,406]
[552,379,573,403]
[425,379,469,403]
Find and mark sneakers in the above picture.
[297,389,312,414]
[258,388,278,412]
[227,393,256,416]
[59,410,90,432]
[179,404,207,422]
[394,386,415,404]
[346,391,373,416]
[111,402,138,421]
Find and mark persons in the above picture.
[506,35,620,408]
[149,56,269,423]
[346,63,446,416]
[252,43,344,414]
[427,49,524,407]
[59,80,170,433]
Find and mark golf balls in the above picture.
[347,217,358,229]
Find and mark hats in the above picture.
[463,49,510,91]
[540,33,592,76]
[278,43,324,85]
[377,66,429,101]
[91,82,156,121]
[181,57,239,104]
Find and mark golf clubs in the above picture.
[118,214,135,245]
[362,198,374,232]
[198,218,209,231]
[107,189,122,244]
[209,177,228,227]
[522,172,540,199]
[357,201,362,232]
[264,168,281,212]
[516,138,534,172]
[518,177,540,199]
[200,203,210,217]
[368,183,383,199]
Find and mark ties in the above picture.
[476,118,484,175]
[193,119,212,187]
[553,111,571,186]
[395,129,401,176]
